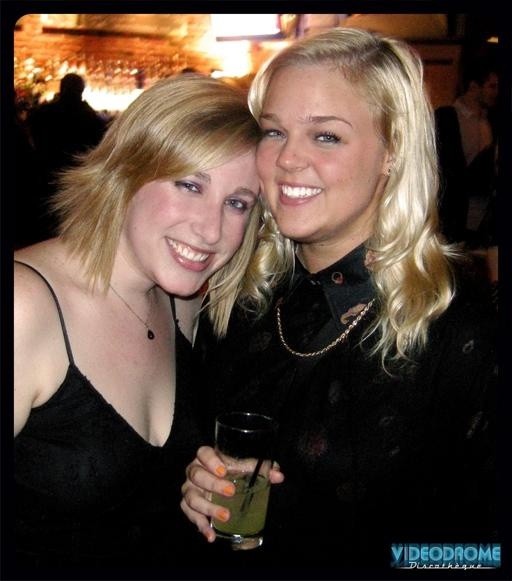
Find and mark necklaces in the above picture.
[108,280,155,339]
[275,297,377,357]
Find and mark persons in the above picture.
[179,23,498,547]
[12,71,265,530]
[24,72,107,148]
[451,60,498,231]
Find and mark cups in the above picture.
[210,413,273,550]
